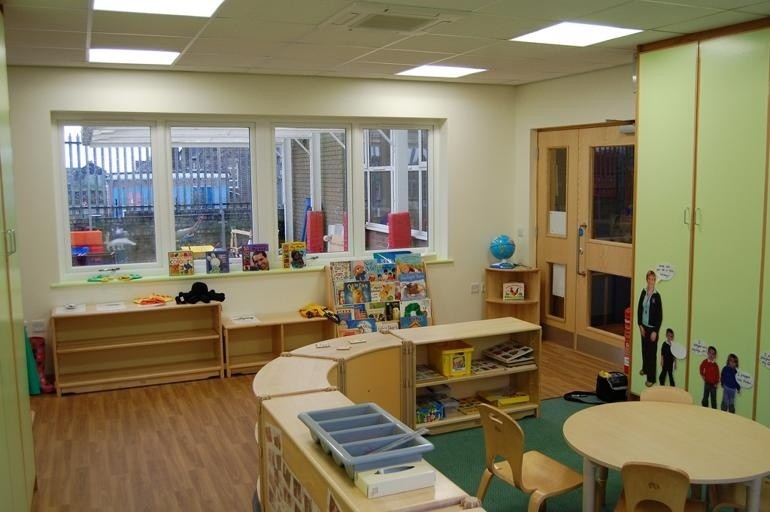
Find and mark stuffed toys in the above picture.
[175,282,226,305]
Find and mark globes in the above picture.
[489,235,515,269]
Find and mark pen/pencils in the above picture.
[374,465,415,475]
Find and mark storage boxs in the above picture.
[425,340,474,378]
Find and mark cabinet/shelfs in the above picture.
[753,182,769,431]
[391,316,539,435]
[51,299,225,396]
[0,12,37,512]
[258,390,467,512]
[628,23,767,419]
[288,332,400,422]
[223,314,336,380]
[252,356,338,512]
[486,267,541,326]
[326,259,434,338]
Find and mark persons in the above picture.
[252,251,269,270]
[637,270,662,387]
[720,354,741,414]
[699,346,720,409]
[659,328,678,387]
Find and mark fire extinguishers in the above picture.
[624,307,632,374]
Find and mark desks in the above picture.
[562,402,770,511]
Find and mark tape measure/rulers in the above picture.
[365,427,429,456]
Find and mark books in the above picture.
[168,251,194,275]
[456,386,489,416]
[282,242,307,269]
[242,244,270,271]
[330,252,432,337]
[471,339,535,375]
[205,251,230,273]
[416,366,448,382]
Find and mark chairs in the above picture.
[639,387,692,403]
[615,463,706,512]
[476,403,602,512]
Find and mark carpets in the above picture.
[419,395,717,512]
